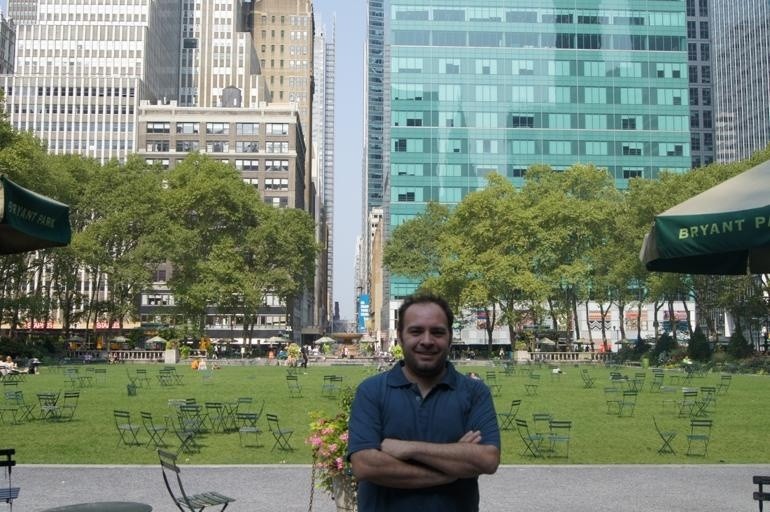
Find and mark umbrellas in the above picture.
[638,157,770,275]
[218,338,239,344]
[265,334,295,345]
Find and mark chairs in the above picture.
[114,398,294,453]
[753,476,770,512]
[0,389,79,425]
[127,366,184,389]
[486,362,541,397]
[605,368,732,458]
[0,366,27,388]
[330,377,344,399]
[496,400,572,458]
[322,375,336,395]
[64,368,108,389]
[286,375,303,398]
[157,451,235,512]
[579,368,596,389]
[0,448,20,512]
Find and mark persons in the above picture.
[0,355,6,377]
[349,298,502,512]
[84,352,91,364]
[4,356,30,375]
